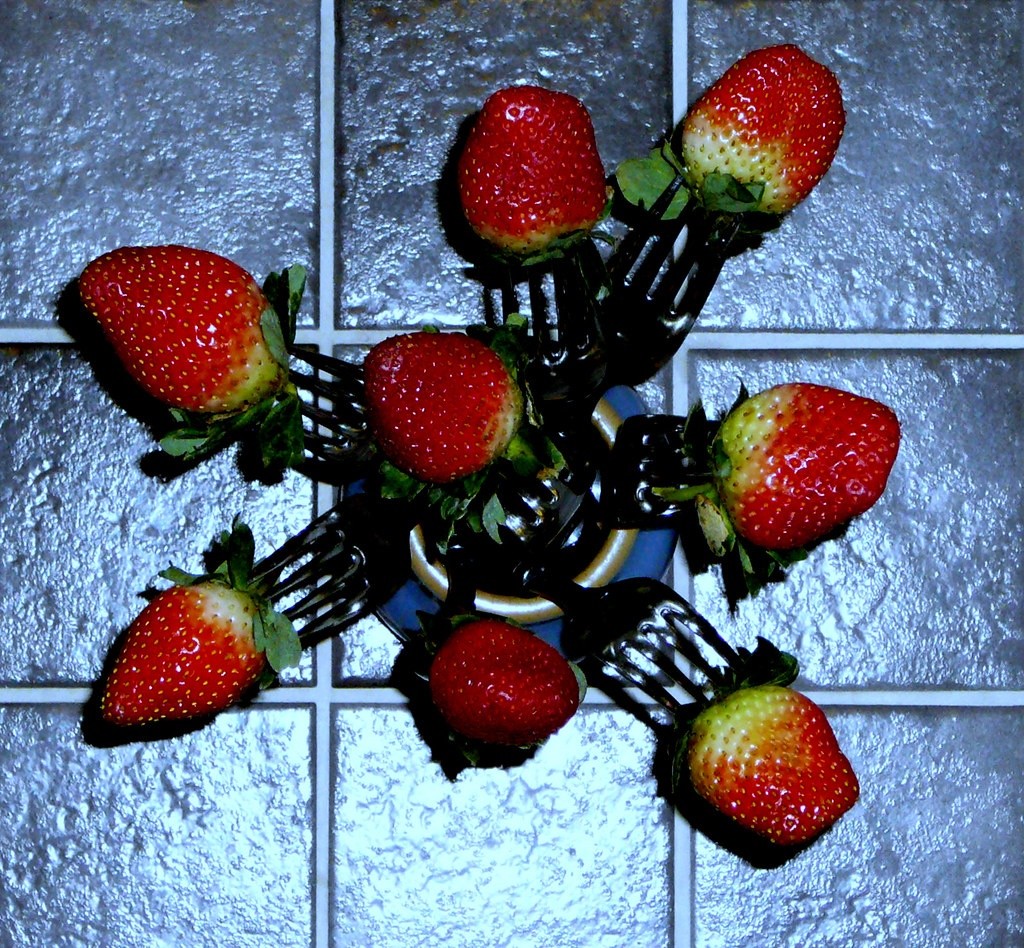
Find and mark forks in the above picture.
[230,175,748,737]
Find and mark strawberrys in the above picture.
[79,42,900,846]
